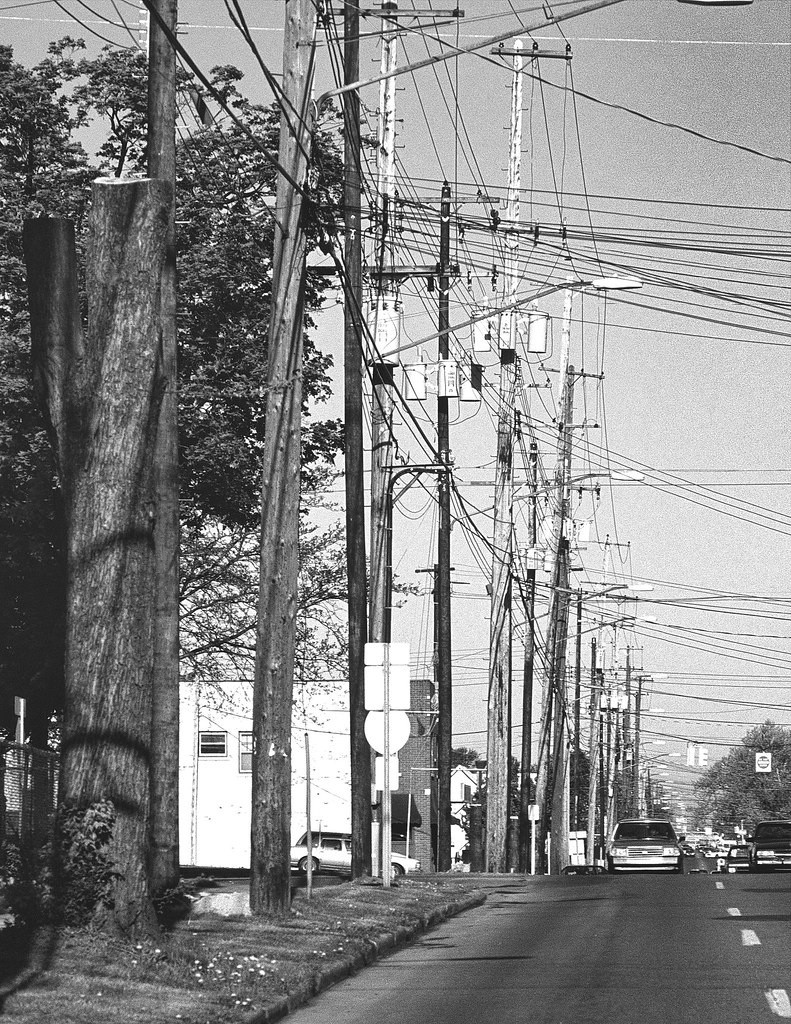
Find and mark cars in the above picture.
[608,819,686,875]
[746,820,791,871]
[289,838,408,880]
[296,832,421,871]
[682,834,738,856]
[720,845,750,873]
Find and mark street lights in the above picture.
[488,582,680,871]
[345,275,645,882]
[438,468,644,872]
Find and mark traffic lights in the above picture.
[700,748,708,765]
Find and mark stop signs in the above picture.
[756,753,771,773]
